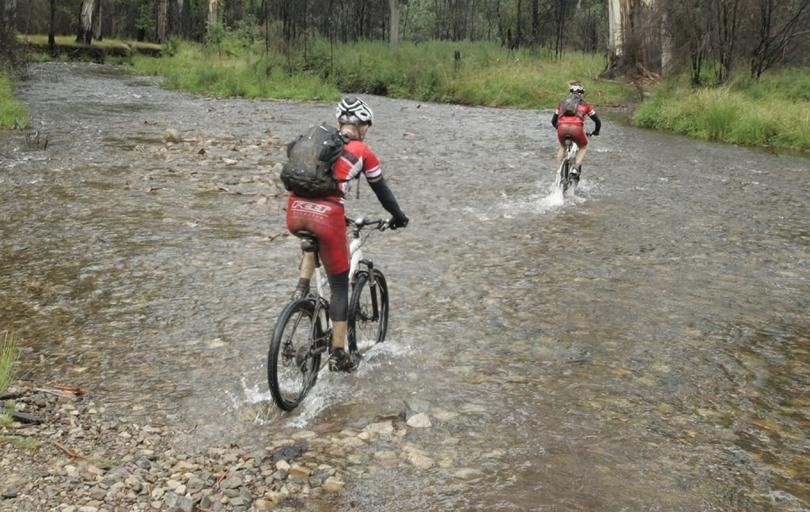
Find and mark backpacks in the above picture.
[278,120,364,201]
[561,94,584,117]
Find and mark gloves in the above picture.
[387,212,410,231]
[591,130,599,136]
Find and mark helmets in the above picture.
[334,93,375,128]
[569,82,585,94]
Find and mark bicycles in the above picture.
[267,211,408,413]
[557,130,599,199]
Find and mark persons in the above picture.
[284,92,408,371]
[550,80,603,181]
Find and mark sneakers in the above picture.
[289,287,309,307]
[328,348,358,373]
[570,166,582,177]
